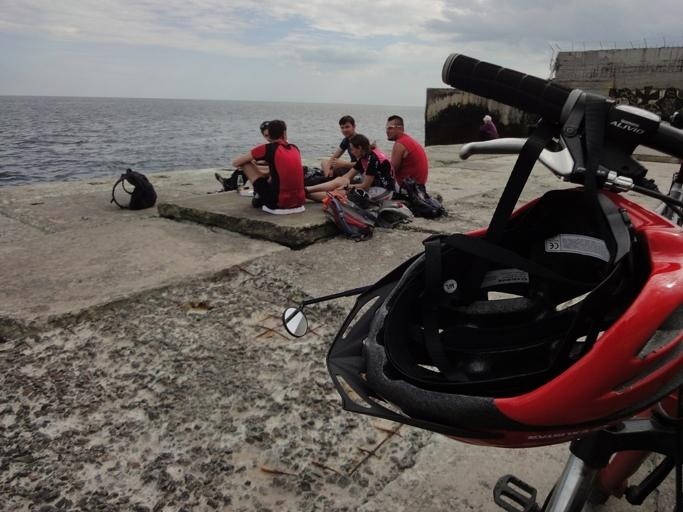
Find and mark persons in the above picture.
[302,134,395,203]
[320,116,370,184]
[230,118,304,208]
[478,115,498,142]
[383,116,429,189]
[214,120,272,192]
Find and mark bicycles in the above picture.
[440,49,683,510]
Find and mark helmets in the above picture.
[326,181,682,450]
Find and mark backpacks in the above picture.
[322,176,444,242]
[109,168,157,211]
